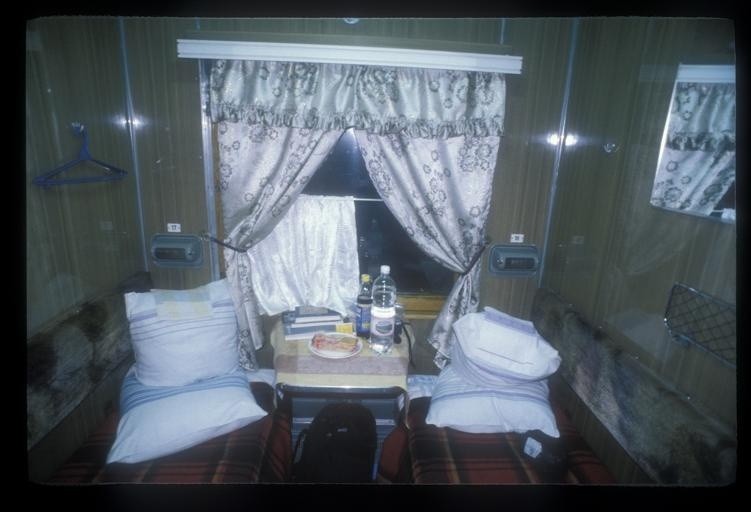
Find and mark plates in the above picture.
[308,332,364,360]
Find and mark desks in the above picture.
[274,316,408,402]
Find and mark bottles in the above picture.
[372,264,398,355]
[356,273,372,339]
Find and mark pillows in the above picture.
[449,312,562,380]
[123,277,239,388]
[425,362,562,439]
[106,364,267,464]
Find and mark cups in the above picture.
[334,322,355,332]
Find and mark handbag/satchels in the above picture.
[520,429,570,483]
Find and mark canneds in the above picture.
[394,303,404,319]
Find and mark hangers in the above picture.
[32,126,127,189]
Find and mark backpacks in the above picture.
[290,403,378,483]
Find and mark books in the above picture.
[280,306,355,340]
